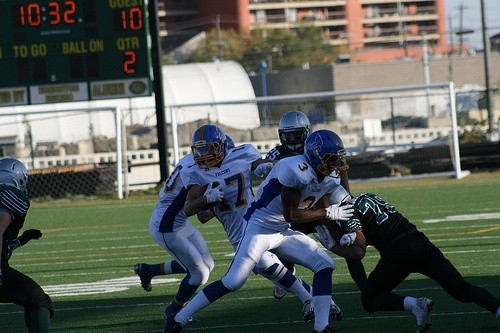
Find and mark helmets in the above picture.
[318,182,350,211]
[191,125,225,168]
[304,130,346,180]
[0,157,28,191]
[223,136,234,149]
[278,112,310,153]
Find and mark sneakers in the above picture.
[165,304,193,321]
[273,267,295,299]
[134,263,152,291]
[413,298,435,333]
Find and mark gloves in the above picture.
[19,229,42,245]
[326,204,354,221]
[313,224,337,250]
[204,181,224,203]
[254,162,273,178]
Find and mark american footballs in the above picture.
[199,181,222,210]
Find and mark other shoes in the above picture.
[303,299,315,322]
[314,326,337,333]
[164,314,179,333]
[329,298,343,320]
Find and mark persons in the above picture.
[0,158,55,333]
[134,111,500,333]
